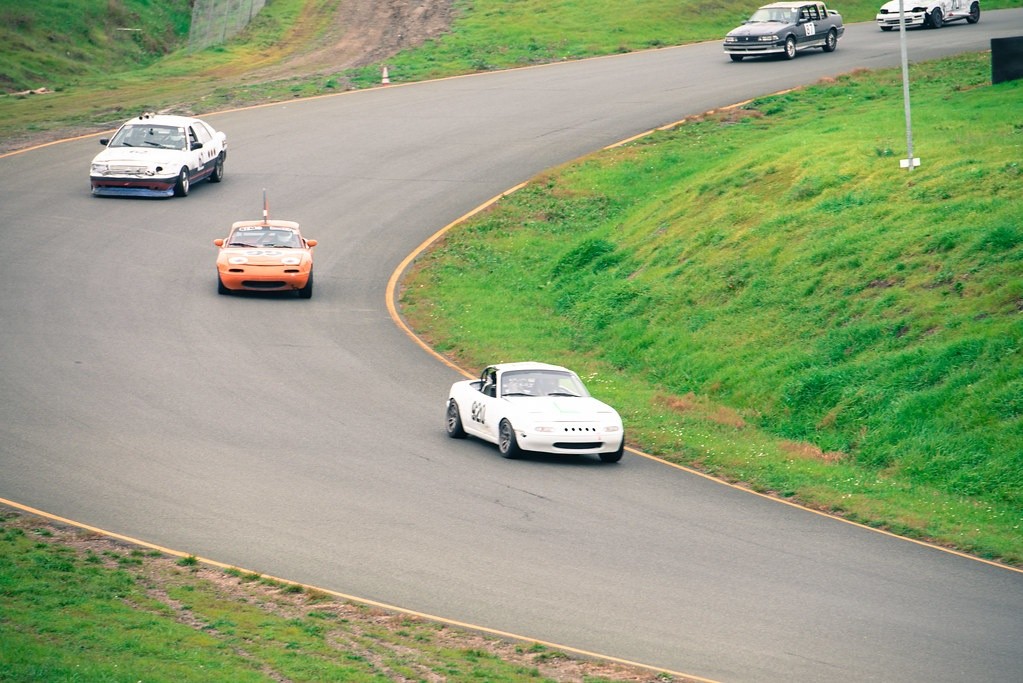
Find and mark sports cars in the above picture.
[214,220,317,300]
[89,113,227,198]
[444,361,624,465]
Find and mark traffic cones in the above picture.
[381,66,390,86]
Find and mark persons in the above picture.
[275,231,296,247]
[165,131,185,149]
[535,379,566,394]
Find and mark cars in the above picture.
[876,0,981,32]
[723,1,844,62]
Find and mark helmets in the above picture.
[171,128,181,141]
[277,232,292,241]
